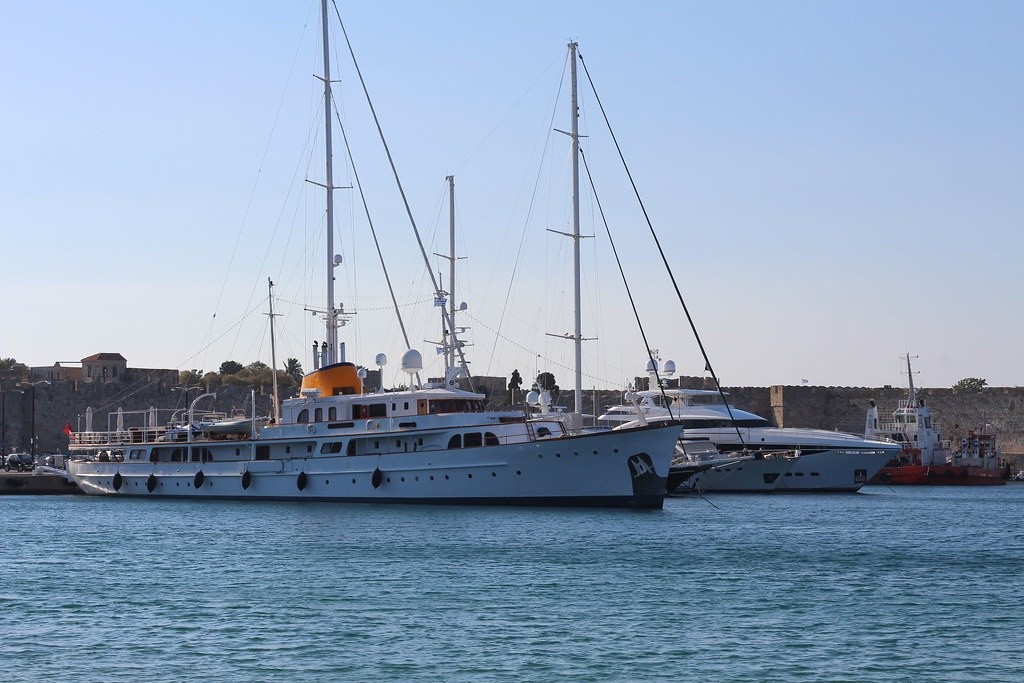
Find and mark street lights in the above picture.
[170,387,205,426]
[16,380,51,461]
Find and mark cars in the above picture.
[4,454,35,472]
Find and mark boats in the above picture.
[951,427,1011,486]
[864,352,956,485]
[598,347,904,494]
[199,417,270,437]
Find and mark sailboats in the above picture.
[64,1,685,511]
[482,38,802,495]
